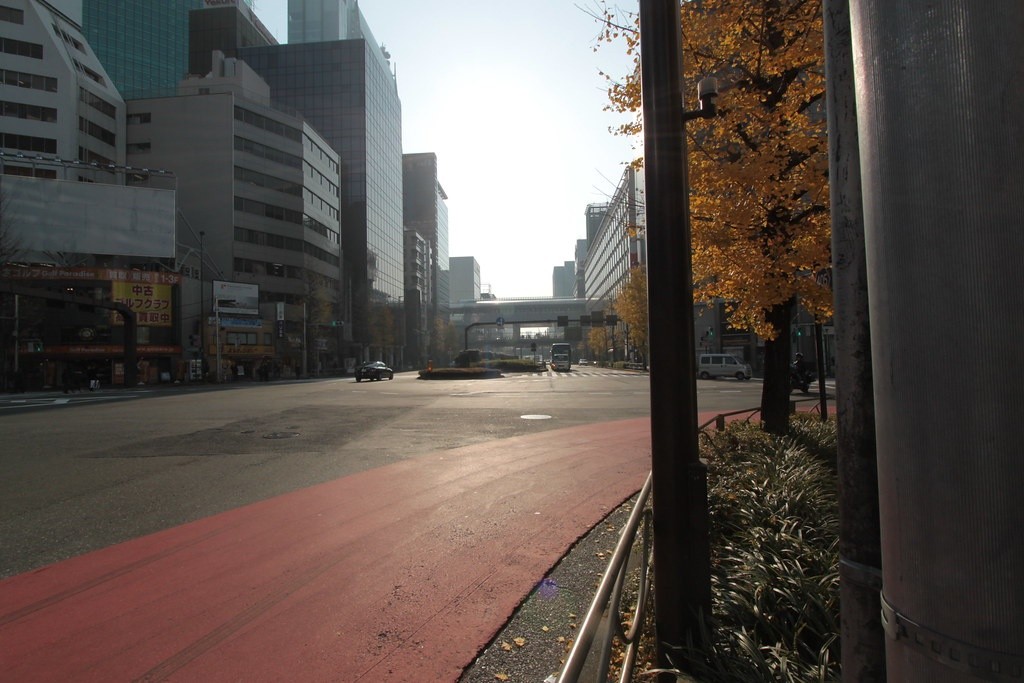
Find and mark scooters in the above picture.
[789,366,816,393]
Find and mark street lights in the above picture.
[199,229,207,385]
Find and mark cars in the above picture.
[354,360,393,382]
[579,358,588,367]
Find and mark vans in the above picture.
[699,354,752,381]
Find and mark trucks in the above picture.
[553,353,569,372]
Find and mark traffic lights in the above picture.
[332,321,344,326]
[709,326,714,337]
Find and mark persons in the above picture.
[794,352,809,383]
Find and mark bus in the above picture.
[550,343,571,370]
